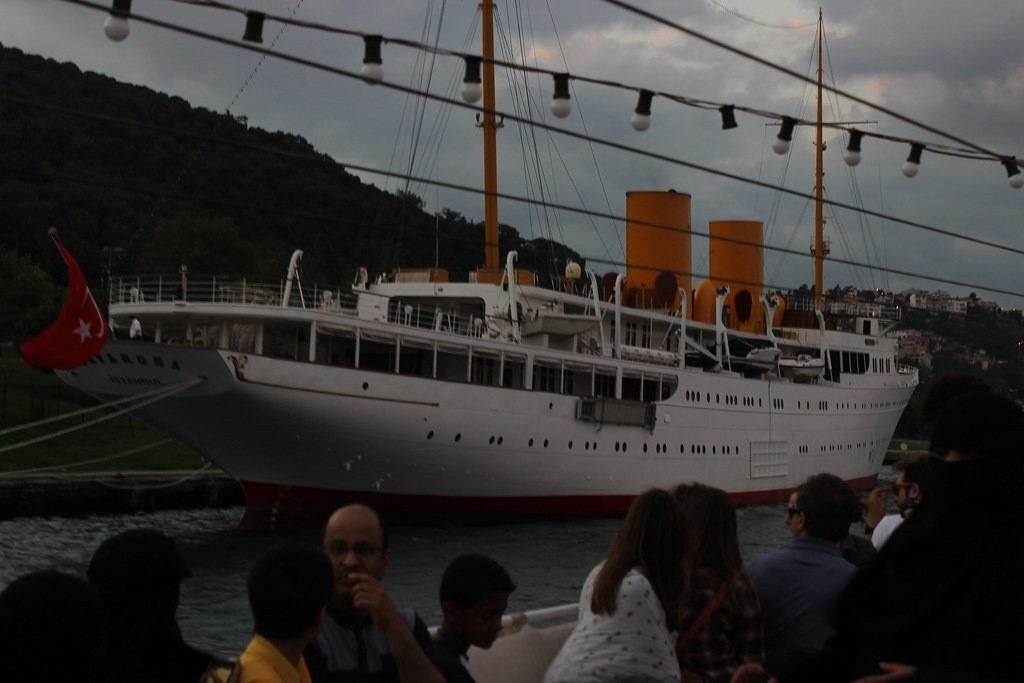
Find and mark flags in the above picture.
[21,234,111,370]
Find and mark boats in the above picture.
[615,342,676,366]
[493,304,601,344]
[777,355,824,378]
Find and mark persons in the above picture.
[432,553,517,683]
[784,486,877,570]
[239,543,335,683]
[748,473,869,683]
[543,488,689,683]
[303,504,446,683]
[864,454,944,552]
[674,483,766,683]
[129,315,142,340]
[0,528,237,683]
[830,373,1024,683]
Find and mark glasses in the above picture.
[786,506,801,517]
[891,482,917,496]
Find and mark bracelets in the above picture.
[864,526,874,535]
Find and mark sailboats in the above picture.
[23,0,923,535]
